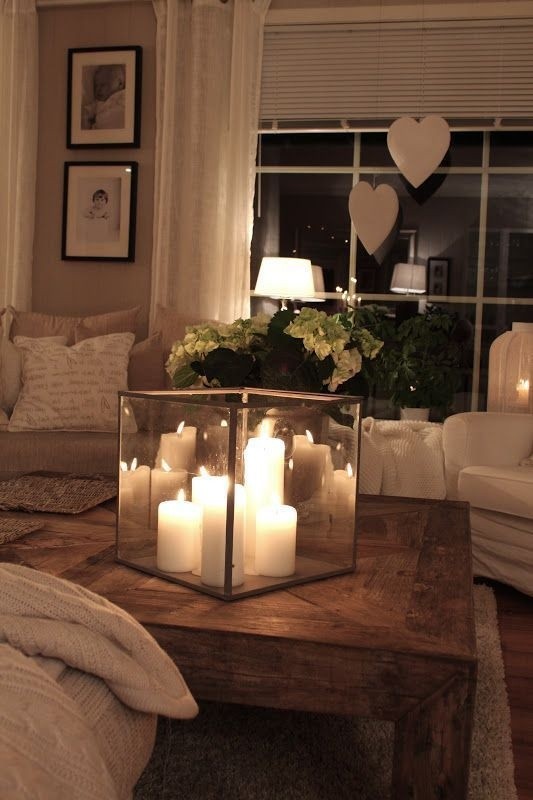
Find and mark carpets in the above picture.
[127,586,515,800]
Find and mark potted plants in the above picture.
[395,310,461,422]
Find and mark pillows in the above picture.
[0,303,163,433]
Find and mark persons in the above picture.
[84,188,110,220]
[80,65,125,129]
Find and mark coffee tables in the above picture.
[0,470,479,800]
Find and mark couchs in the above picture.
[0,313,217,477]
[439,407,533,598]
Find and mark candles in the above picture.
[516,380,529,403]
[117,420,352,591]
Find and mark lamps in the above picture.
[392,264,427,293]
[292,266,325,313]
[258,257,314,314]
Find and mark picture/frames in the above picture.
[61,160,138,262]
[65,45,142,148]
[427,257,450,298]
